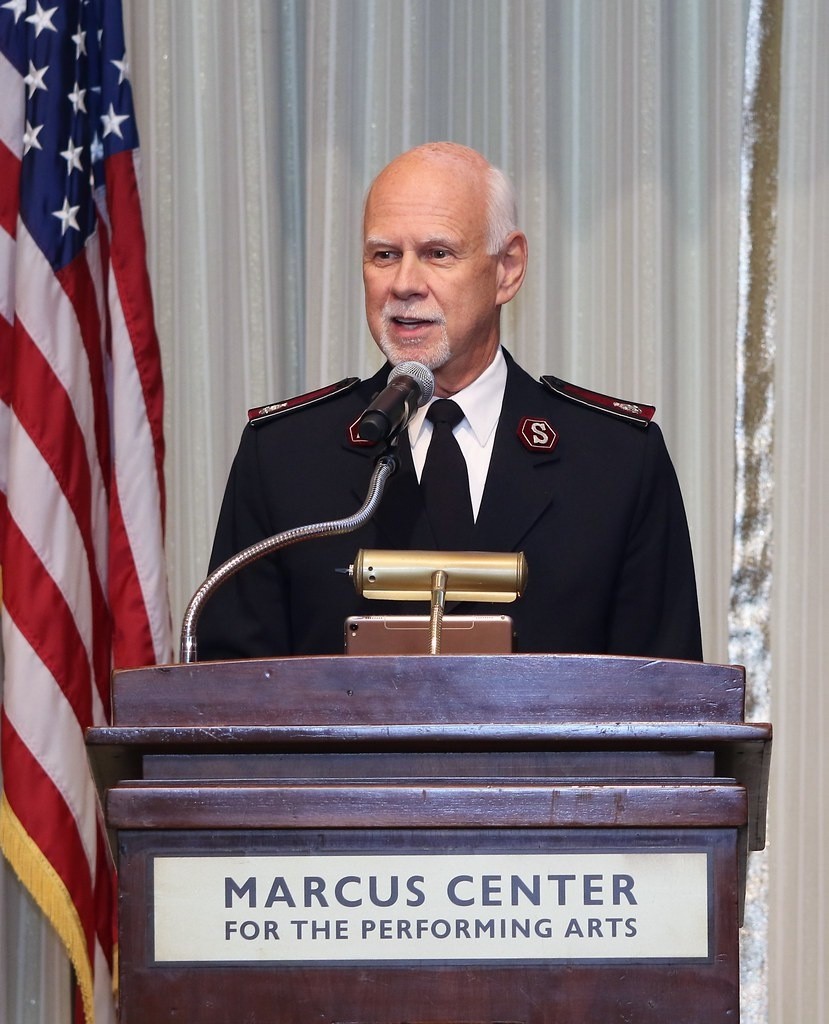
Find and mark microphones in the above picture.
[358,361,434,441]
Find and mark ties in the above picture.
[409,399,474,552]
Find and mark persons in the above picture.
[195,141,703,663]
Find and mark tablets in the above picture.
[345,615,514,658]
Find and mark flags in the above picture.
[0,0,168,1023]
